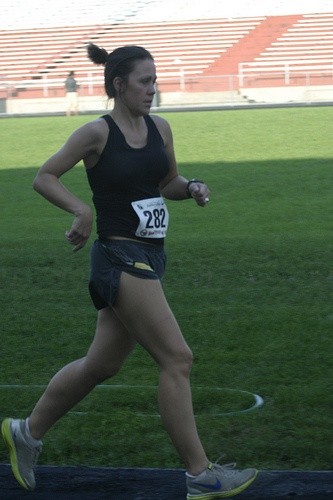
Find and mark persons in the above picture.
[64,70,80,116]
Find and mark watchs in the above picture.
[1,43,259,500]
[185,178,205,199]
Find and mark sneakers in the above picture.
[185,456,258,500]
[2,417,43,491]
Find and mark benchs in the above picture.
[0,14,333,96]
